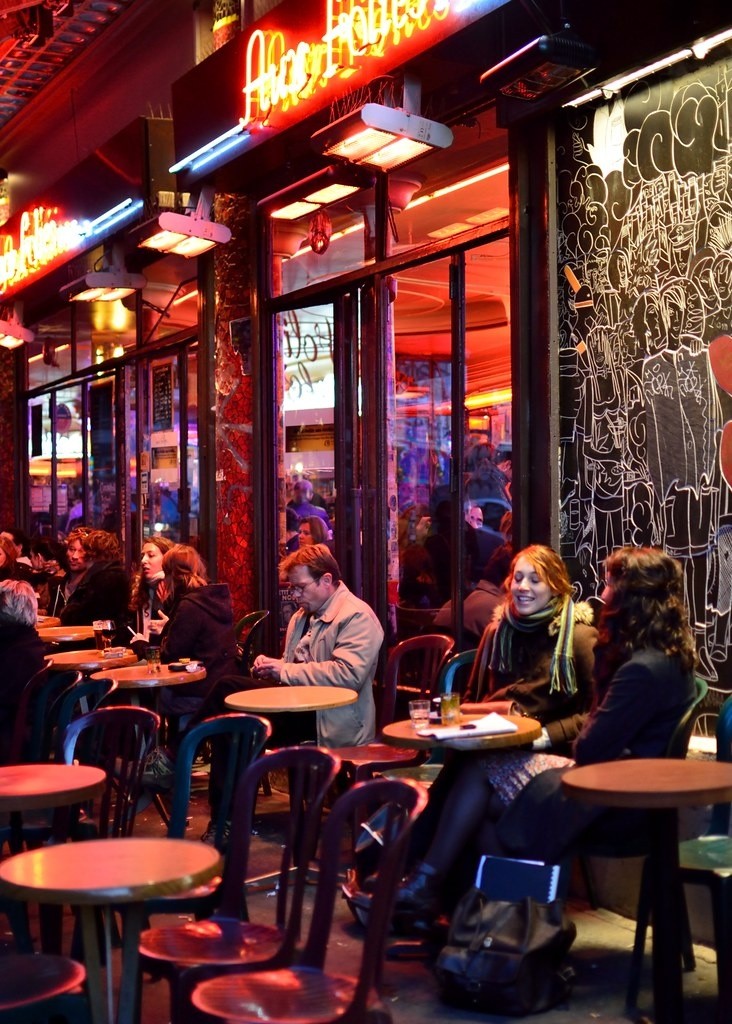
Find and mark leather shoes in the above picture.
[348,867,450,920]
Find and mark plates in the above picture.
[427,710,457,722]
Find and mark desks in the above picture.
[382,715,542,775]
[0,837,223,1024]
[562,755,732,1024]
[224,685,359,713]
[33,614,207,689]
[0,763,107,853]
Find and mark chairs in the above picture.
[439,648,600,911]
[141,712,272,965]
[624,695,732,1024]
[234,610,272,796]
[0,659,160,1024]
[576,677,708,974]
[134,745,342,1024]
[307,634,455,859]
[190,776,429,1024]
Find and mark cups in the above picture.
[440,692,462,728]
[406,699,431,729]
[433,697,443,716]
[93,620,102,650]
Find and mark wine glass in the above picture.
[101,619,118,657]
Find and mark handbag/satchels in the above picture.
[459,700,527,718]
[437,886,578,1016]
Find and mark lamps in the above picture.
[59,272,147,302]
[480,24,593,103]
[310,103,454,175]
[0,319,34,350]
[128,212,232,259]
[257,164,374,223]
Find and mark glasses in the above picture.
[72,528,89,537]
[290,579,318,594]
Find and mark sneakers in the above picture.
[128,745,175,794]
[201,818,234,853]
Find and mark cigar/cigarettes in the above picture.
[127,626,136,636]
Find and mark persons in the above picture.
[352,546,696,961]
[0,442,515,860]
[342,545,601,930]
